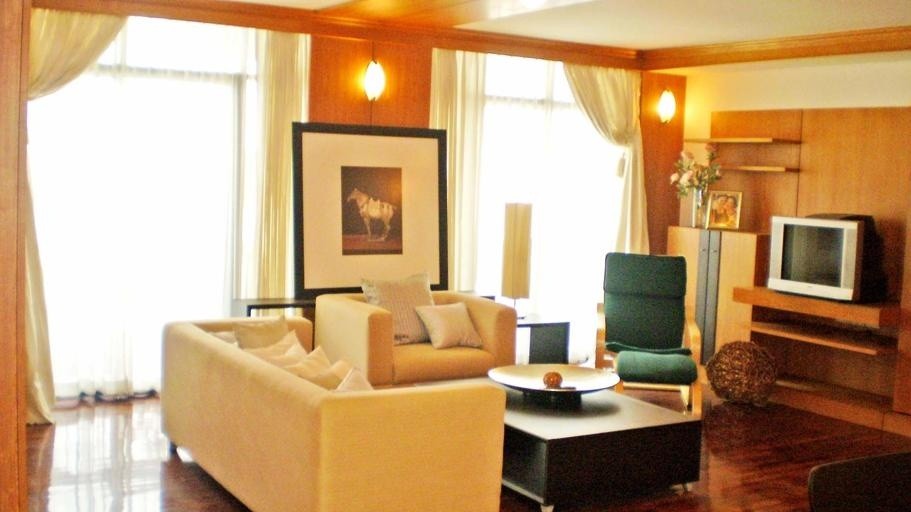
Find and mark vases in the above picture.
[692,185,713,230]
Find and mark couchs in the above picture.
[160,314,506,512]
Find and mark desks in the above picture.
[243,295,495,317]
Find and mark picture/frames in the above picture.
[291,121,448,301]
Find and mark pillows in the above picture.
[231,270,482,391]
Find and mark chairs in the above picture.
[313,290,517,389]
[595,251,704,417]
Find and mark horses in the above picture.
[347,189,398,243]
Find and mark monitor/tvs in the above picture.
[767,211,888,302]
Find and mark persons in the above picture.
[722,196,737,225]
[711,195,728,224]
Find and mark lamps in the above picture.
[658,88,676,124]
[364,57,385,102]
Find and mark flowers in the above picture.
[670,151,723,207]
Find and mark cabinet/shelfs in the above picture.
[681,137,799,173]
[731,286,898,358]
[666,227,787,386]
